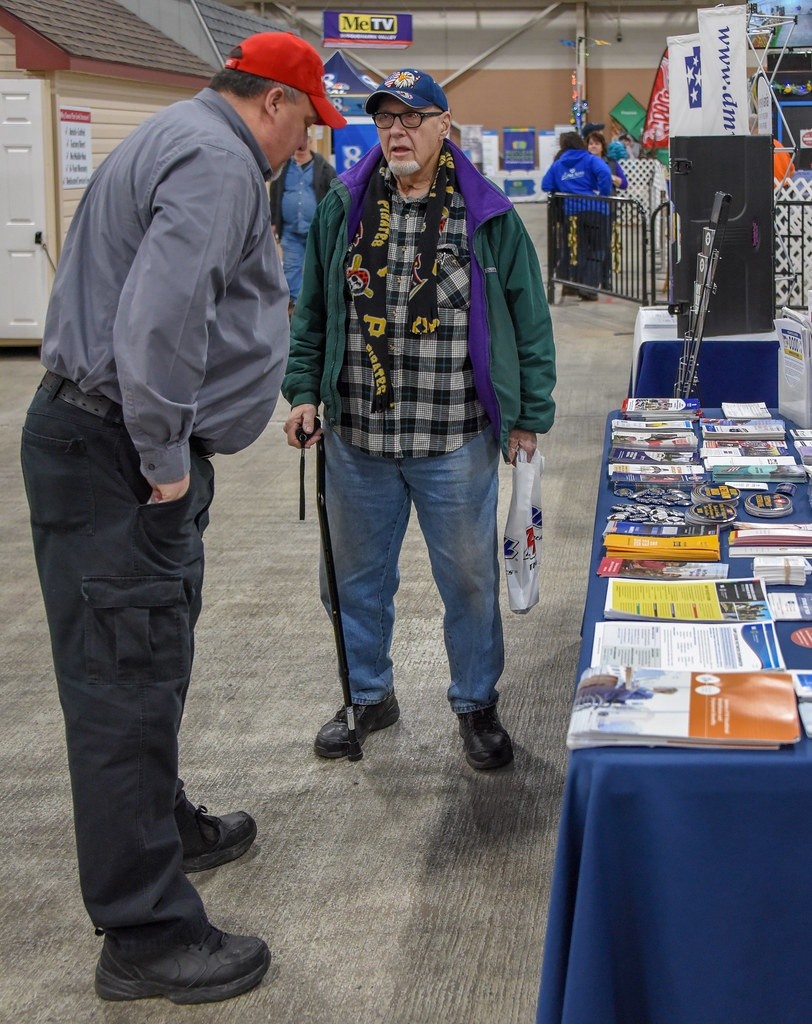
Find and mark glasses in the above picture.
[372,112,442,130]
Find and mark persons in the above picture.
[21,30,350,1008]
[580,123,628,294]
[541,132,612,302]
[282,68,556,772]
[608,134,632,160]
[270,138,338,319]
[599,667,678,699]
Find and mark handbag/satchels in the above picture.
[502,447,545,614]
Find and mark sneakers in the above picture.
[91,921,271,1003]
[456,704,513,769]
[314,688,400,757]
[181,804,257,871]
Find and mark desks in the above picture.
[535,406,812,1024]
[624,306,778,406]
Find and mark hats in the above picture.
[224,31,346,128]
[582,122,605,137]
[619,133,635,146]
[365,68,448,116]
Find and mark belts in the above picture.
[41,370,215,459]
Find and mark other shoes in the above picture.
[564,286,578,295]
[578,289,600,302]
